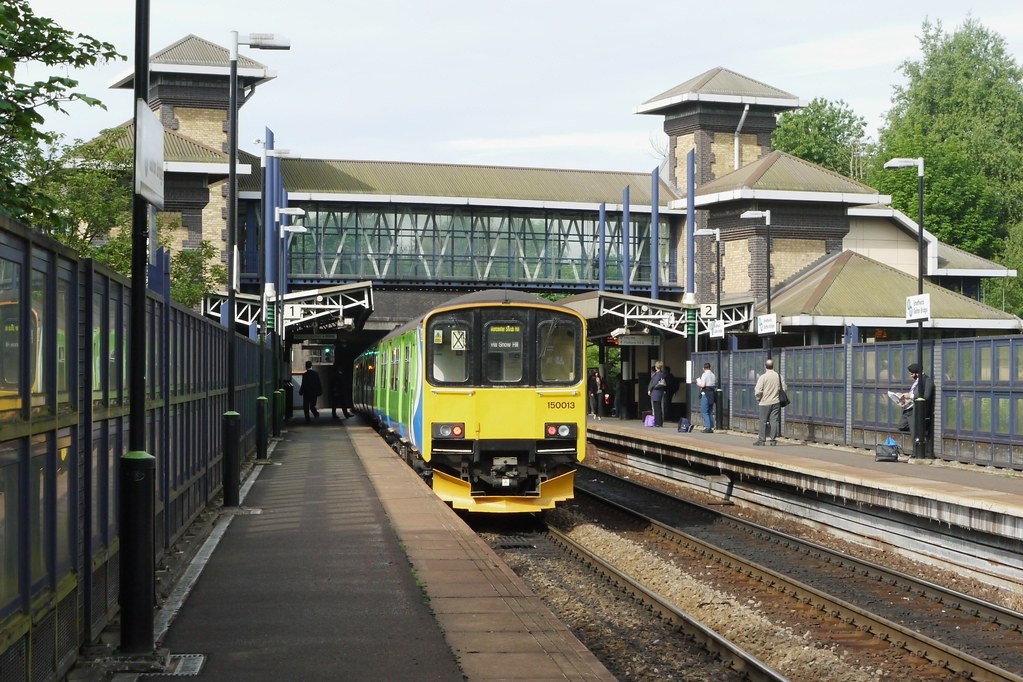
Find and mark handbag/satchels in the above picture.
[678,417,689,432]
[779,375,788,407]
[645,415,655,426]
[600,378,606,390]
[655,370,666,389]
[876,443,904,462]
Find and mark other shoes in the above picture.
[770,442,776,446]
[701,428,713,433]
[315,413,319,423]
[598,416,602,421]
[594,413,597,419]
[346,414,354,418]
[753,441,764,446]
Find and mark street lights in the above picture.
[220,29,291,510]
[271,204,308,442]
[740,209,770,438]
[253,145,292,466]
[693,228,723,431]
[884,156,926,459]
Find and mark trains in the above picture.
[350,286,590,528]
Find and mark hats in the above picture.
[908,364,923,373]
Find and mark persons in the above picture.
[697,363,716,433]
[328,359,355,419]
[586,368,606,420]
[648,361,667,428]
[299,361,322,425]
[753,360,780,446]
[896,363,934,459]
